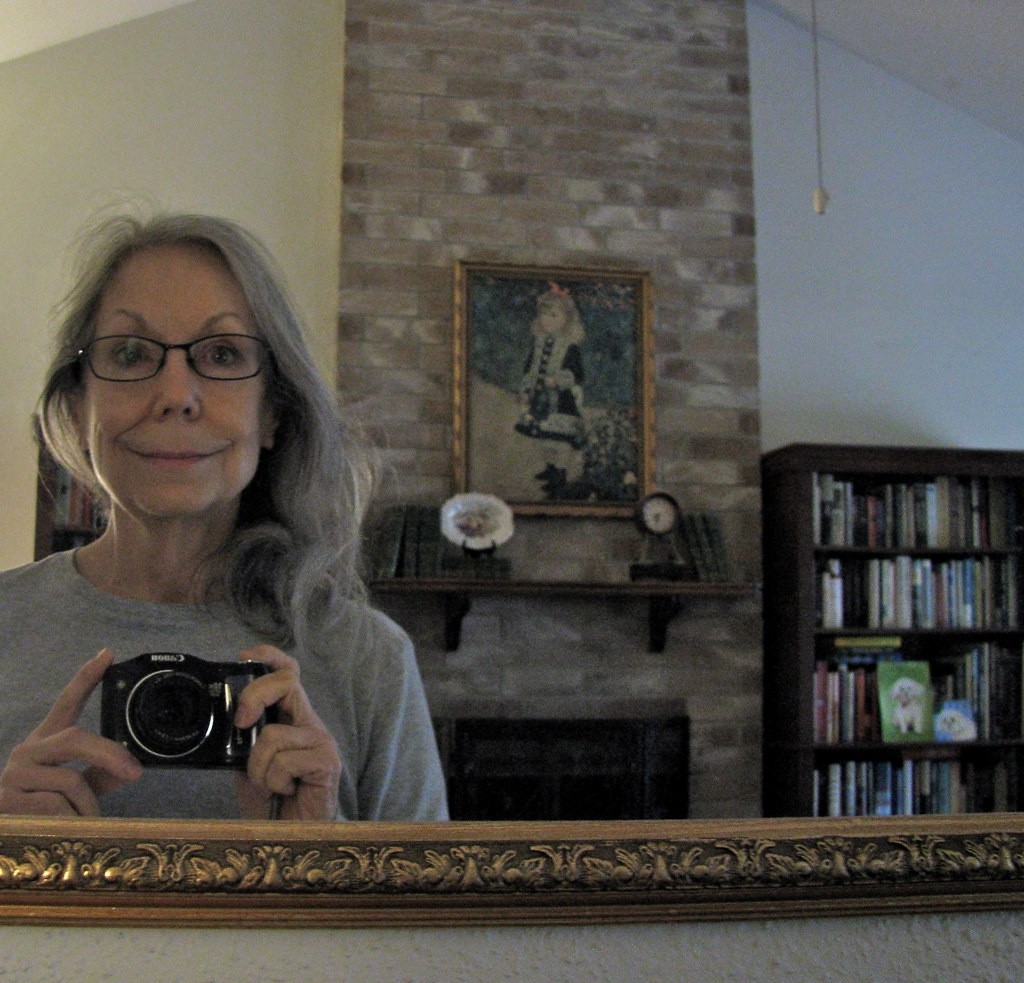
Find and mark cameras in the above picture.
[101,651,279,770]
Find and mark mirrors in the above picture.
[0,0,1024,924]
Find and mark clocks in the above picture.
[633,492,694,581]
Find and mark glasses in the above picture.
[78,333,271,383]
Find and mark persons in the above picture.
[0,203,451,823]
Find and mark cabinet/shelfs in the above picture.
[760,442,1024,818]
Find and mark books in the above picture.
[811,471,1024,819]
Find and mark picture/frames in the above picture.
[452,259,652,519]
